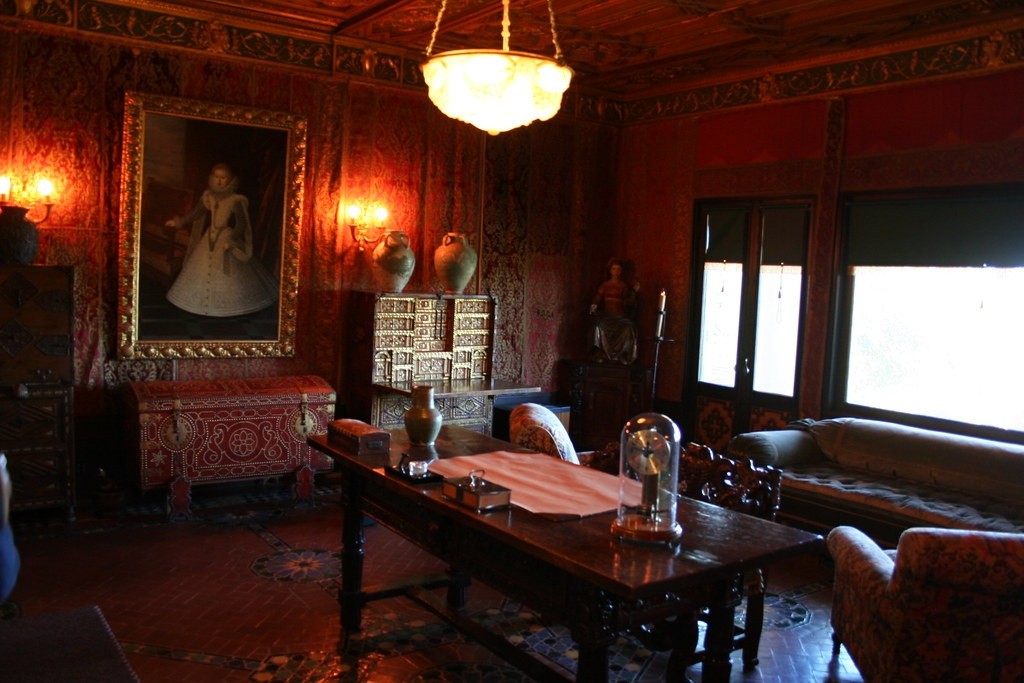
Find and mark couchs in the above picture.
[725,416,1023,547]
[823,524,1023,683]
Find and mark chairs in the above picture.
[589,438,786,683]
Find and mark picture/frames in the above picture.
[115,89,308,359]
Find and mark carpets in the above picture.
[0,605,139,682]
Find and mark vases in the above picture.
[433,232,477,294]
[370,229,415,292]
[0,206,38,264]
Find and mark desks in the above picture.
[307,423,824,683]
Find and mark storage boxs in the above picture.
[121,374,336,519]
[0,264,76,513]
[325,417,391,456]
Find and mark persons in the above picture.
[588,257,642,367]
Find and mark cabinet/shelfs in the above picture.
[555,358,648,452]
[340,287,498,435]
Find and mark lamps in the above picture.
[416,0,577,139]
